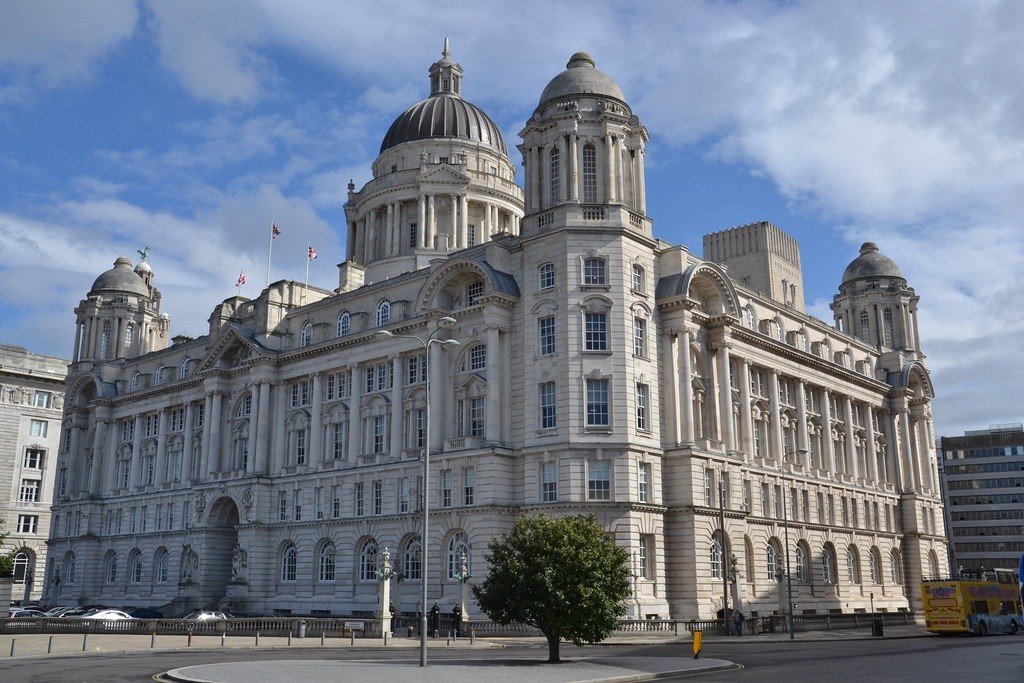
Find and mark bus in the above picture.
[920,566,1024,636]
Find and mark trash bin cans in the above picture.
[872,617,883,636]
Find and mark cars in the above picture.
[9,604,287,628]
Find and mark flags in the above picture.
[235,272,246,286]
[308,246,318,261]
[272,222,283,240]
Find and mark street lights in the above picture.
[781,449,809,639]
[375,315,461,668]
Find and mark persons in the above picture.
[734,608,745,637]
